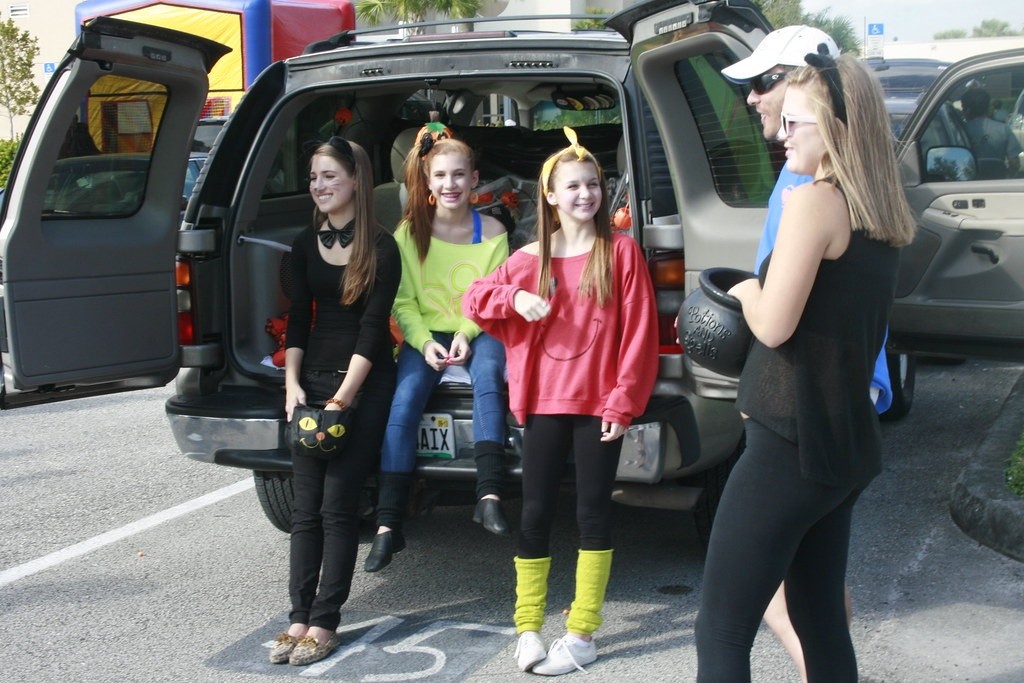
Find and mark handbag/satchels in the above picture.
[281,404,368,461]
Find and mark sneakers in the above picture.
[513,631,546,673]
[533,635,597,676]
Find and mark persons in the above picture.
[266,136,402,666]
[461,125,659,676]
[960,87,1022,180]
[58,114,104,161]
[364,121,512,574]
[672,24,920,683]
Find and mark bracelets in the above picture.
[326,398,347,410]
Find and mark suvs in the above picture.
[0,0,1024,551]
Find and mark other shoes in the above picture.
[270,633,304,664]
[289,631,341,665]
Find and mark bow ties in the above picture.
[318,218,358,250]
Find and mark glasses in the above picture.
[751,72,788,95]
[781,112,819,137]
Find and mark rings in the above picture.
[542,300,548,309]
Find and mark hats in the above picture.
[721,24,840,84]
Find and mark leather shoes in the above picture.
[472,498,512,537]
[364,530,406,572]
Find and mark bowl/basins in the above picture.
[675,267,758,378]
